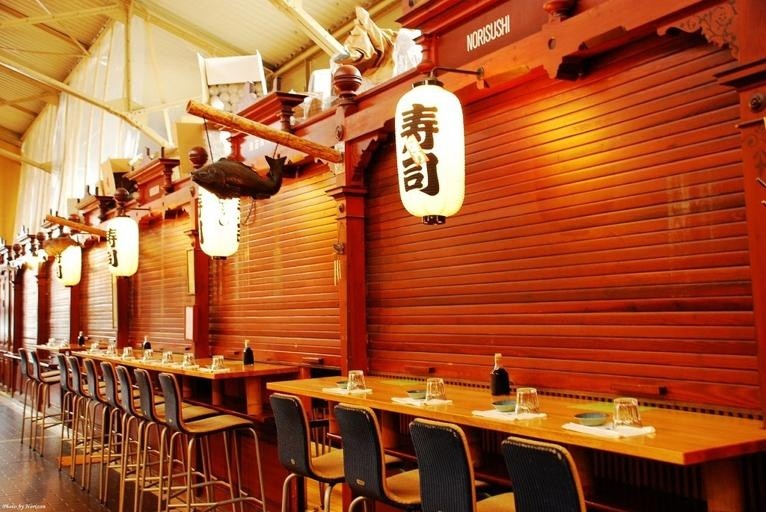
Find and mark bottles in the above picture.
[77,331,84,345]
[143,336,151,348]
[489,352,510,396]
[243,339,254,366]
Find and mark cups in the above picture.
[515,387,541,417]
[613,396,641,432]
[143,349,155,360]
[347,370,366,393]
[183,353,196,366]
[162,351,174,364]
[121,346,135,358]
[426,377,447,402]
[106,345,118,357]
[90,343,100,353]
[211,355,225,370]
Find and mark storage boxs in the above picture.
[100,159,134,196]
[196,49,269,123]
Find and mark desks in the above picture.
[70,346,314,422]
[265,375,766,467]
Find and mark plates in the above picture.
[573,411,610,426]
[491,400,516,412]
[336,381,347,389]
[405,390,426,399]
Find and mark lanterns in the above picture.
[197,185,242,261]
[394,79,466,226]
[56,246,82,287]
[106,215,140,279]
